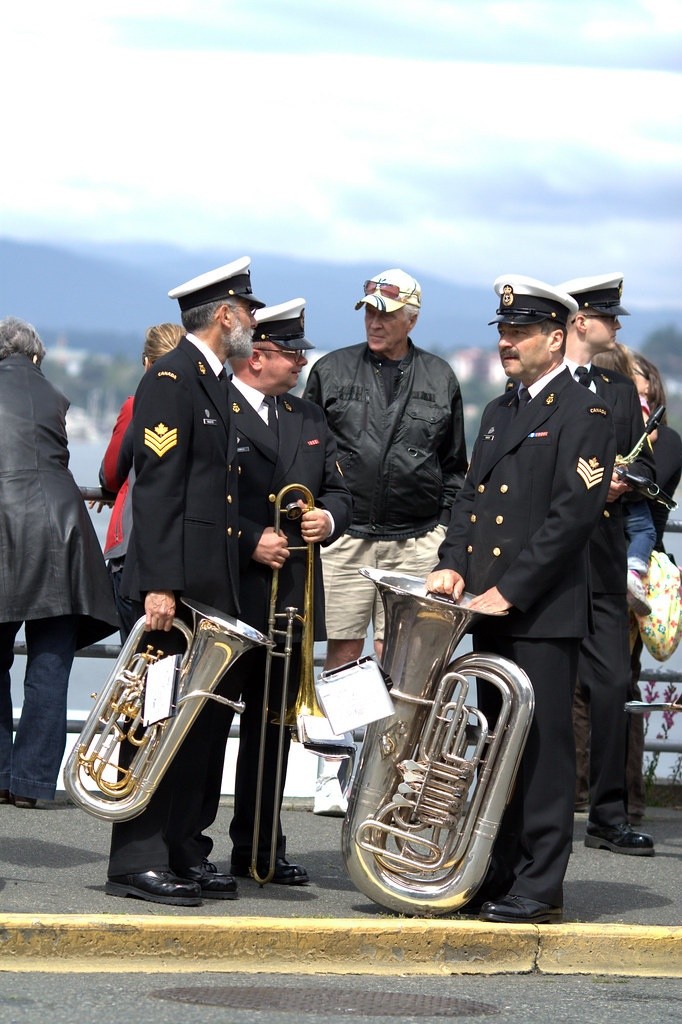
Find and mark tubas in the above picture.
[339,566,538,917]
[62,596,278,823]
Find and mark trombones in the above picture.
[252,482,329,884]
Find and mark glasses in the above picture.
[363,279,420,302]
[142,351,148,365]
[257,348,306,362]
[632,368,649,381]
[571,314,619,325]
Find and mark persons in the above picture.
[104,255,265,908]
[425,273,615,923]
[227,296,353,881]
[0,323,194,808]
[304,266,468,816]
[563,277,682,856]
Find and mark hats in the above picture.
[487,275,579,327]
[168,255,267,309]
[354,266,422,313]
[251,297,317,349]
[553,270,631,315]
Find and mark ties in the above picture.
[264,396,280,438]
[517,388,531,415]
[575,366,591,388]
[219,368,230,402]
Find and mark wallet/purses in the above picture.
[615,466,678,512]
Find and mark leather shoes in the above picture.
[480,893,565,925]
[585,820,656,857]
[230,856,310,884]
[173,857,238,899]
[105,867,202,907]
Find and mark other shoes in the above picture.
[0,790,10,804]
[14,796,37,808]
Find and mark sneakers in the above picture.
[625,569,653,617]
[313,774,348,815]
